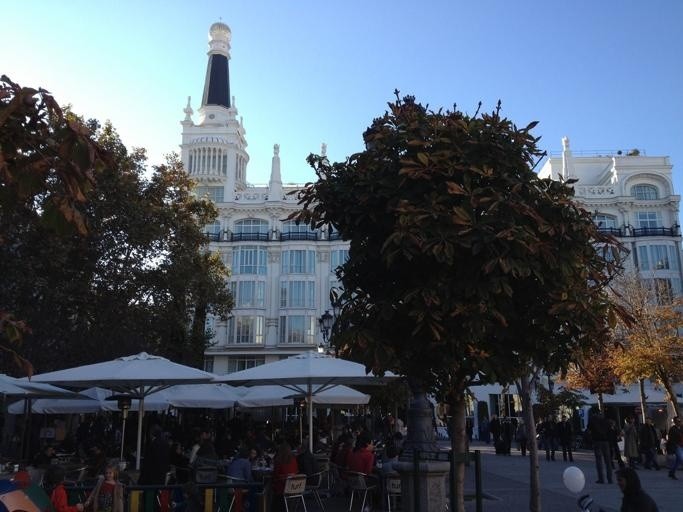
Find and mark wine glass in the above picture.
[253,457,266,468]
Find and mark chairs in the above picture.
[0,451,404,512]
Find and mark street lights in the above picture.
[104,394,144,462]
[317,343,324,354]
[317,309,342,343]
[283,393,317,445]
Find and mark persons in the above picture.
[574,405,683,511]
[2,405,574,511]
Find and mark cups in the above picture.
[13,464,20,473]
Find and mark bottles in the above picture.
[377,458,383,469]
